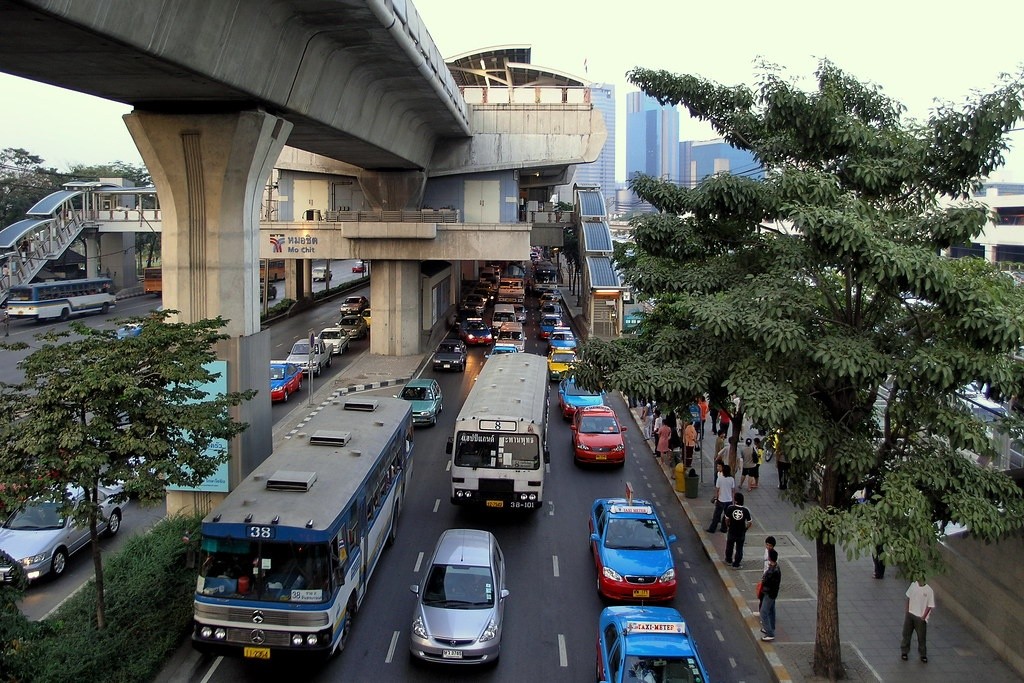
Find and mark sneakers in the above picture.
[761,629,767,634]
[762,635,775,641]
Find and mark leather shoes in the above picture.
[902,653,908,661]
[920,656,927,663]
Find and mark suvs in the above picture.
[432,339,468,373]
[494,321,528,351]
[312,266,333,282]
[393,379,444,427]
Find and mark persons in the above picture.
[706,460,752,570]
[900,570,934,663]
[713,430,788,492]
[627,395,638,408]
[756,536,781,641]
[640,396,745,480]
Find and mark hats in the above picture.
[746,438,752,445]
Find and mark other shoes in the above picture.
[733,565,743,570]
[777,485,782,489]
[720,528,722,531]
[738,485,742,489]
[642,439,649,441]
[698,437,705,441]
[706,529,714,533]
[872,572,875,578]
[653,452,657,455]
[653,455,661,458]
[671,476,676,480]
[725,558,732,566]
[748,488,752,492]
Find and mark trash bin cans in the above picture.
[675,463,687,492]
[684,467,700,499]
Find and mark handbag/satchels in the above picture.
[752,447,758,463]
[695,442,700,452]
[756,581,762,598]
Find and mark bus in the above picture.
[144,266,162,297]
[190,394,416,660]
[6,276,120,325]
[445,352,553,513]
[259,258,285,283]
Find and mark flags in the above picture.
[584,58,589,73]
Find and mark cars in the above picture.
[588,482,679,602]
[317,327,352,356]
[0,432,155,583]
[484,346,519,359]
[558,374,607,422]
[455,317,494,347]
[570,405,628,468]
[260,281,277,303]
[270,359,304,404]
[408,528,511,665]
[352,262,366,273]
[537,316,567,340]
[340,295,370,317]
[453,246,565,335]
[359,307,371,330]
[547,326,579,350]
[596,605,711,683]
[334,315,368,341]
[546,346,582,381]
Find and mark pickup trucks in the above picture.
[284,336,335,378]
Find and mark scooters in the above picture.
[764,426,783,462]
